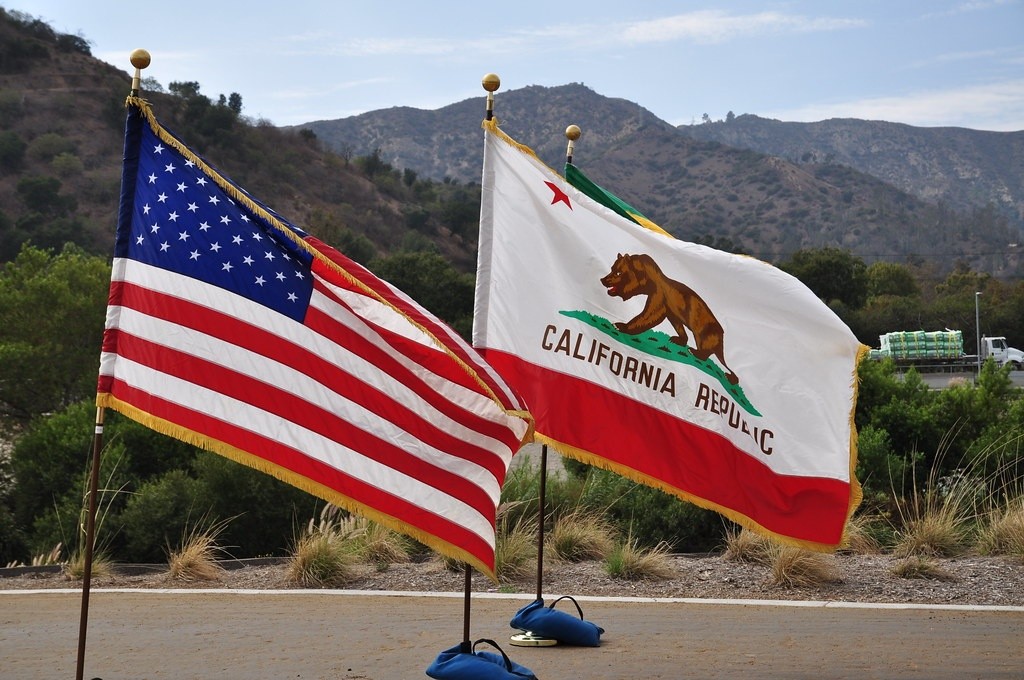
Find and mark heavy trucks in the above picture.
[869,330,1024,371]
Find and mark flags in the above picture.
[95,90,535,589]
[471,116,870,554]
